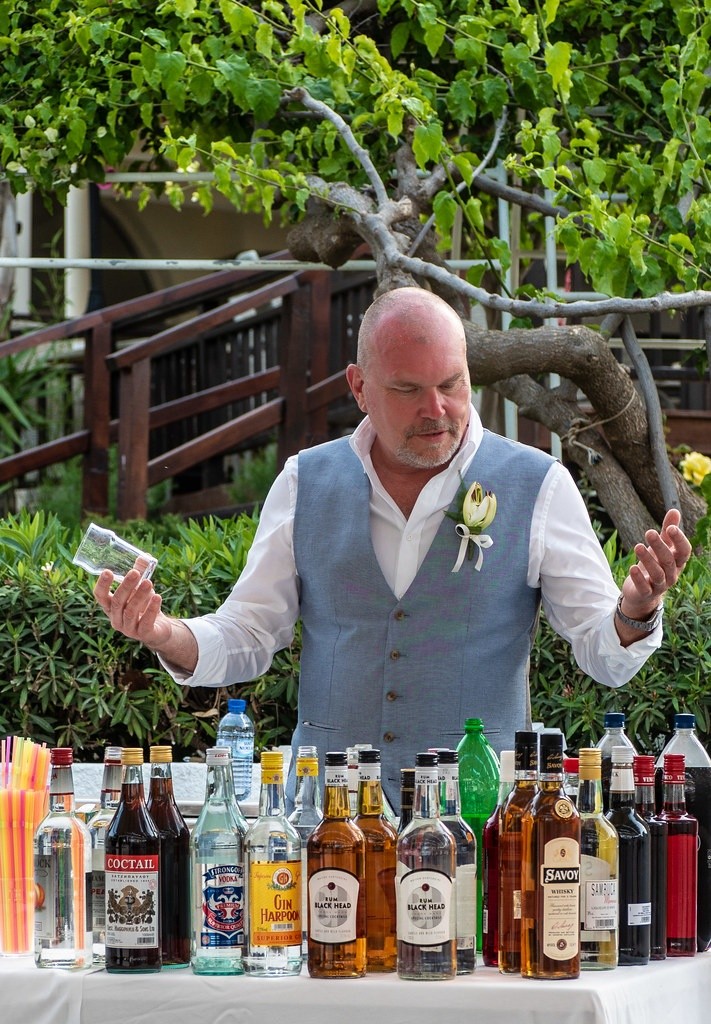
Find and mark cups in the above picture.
[1,878,35,957]
[72,523,159,591]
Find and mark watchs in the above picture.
[616,593,665,631]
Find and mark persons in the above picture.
[92,286,692,814]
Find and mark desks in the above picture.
[0,952,710,1024]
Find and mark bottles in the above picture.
[28,698,711,981]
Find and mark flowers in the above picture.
[442,468,497,561]
